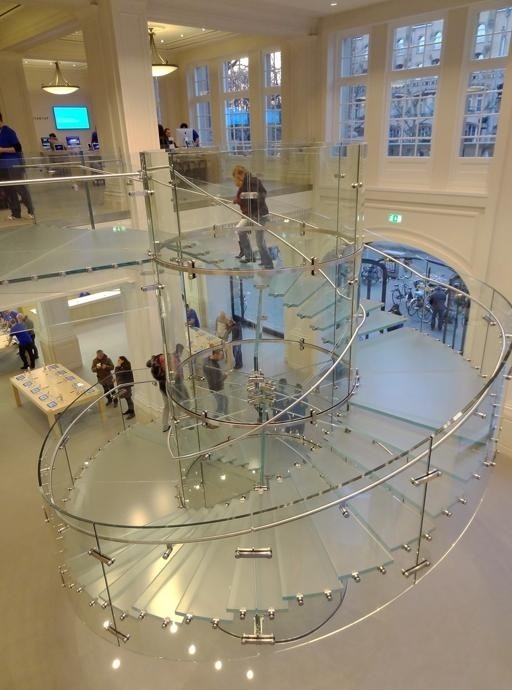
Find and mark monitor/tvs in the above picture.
[175,128,194,147]
[53,106,90,129]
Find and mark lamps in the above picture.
[39,60,79,95]
[145,25,179,76]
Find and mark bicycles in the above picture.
[391,271,433,323]
[361,266,383,286]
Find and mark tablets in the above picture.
[50,364,95,394]
[16,375,57,408]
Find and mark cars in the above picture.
[449,272,467,294]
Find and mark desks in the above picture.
[10,362,109,439]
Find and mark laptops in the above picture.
[40,136,100,150]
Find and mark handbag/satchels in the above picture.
[150,354,165,381]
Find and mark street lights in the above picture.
[468,85,488,157]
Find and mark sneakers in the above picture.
[106,401,135,419]
[235,252,274,269]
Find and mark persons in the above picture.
[429,286,446,330]
[47,133,65,150]
[147,304,312,433]
[0,309,40,370]
[387,304,404,331]
[157,122,200,149]
[232,167,276,272]
[90,349,137,420]
[0,112,36,220]
[88,126,98,145]
